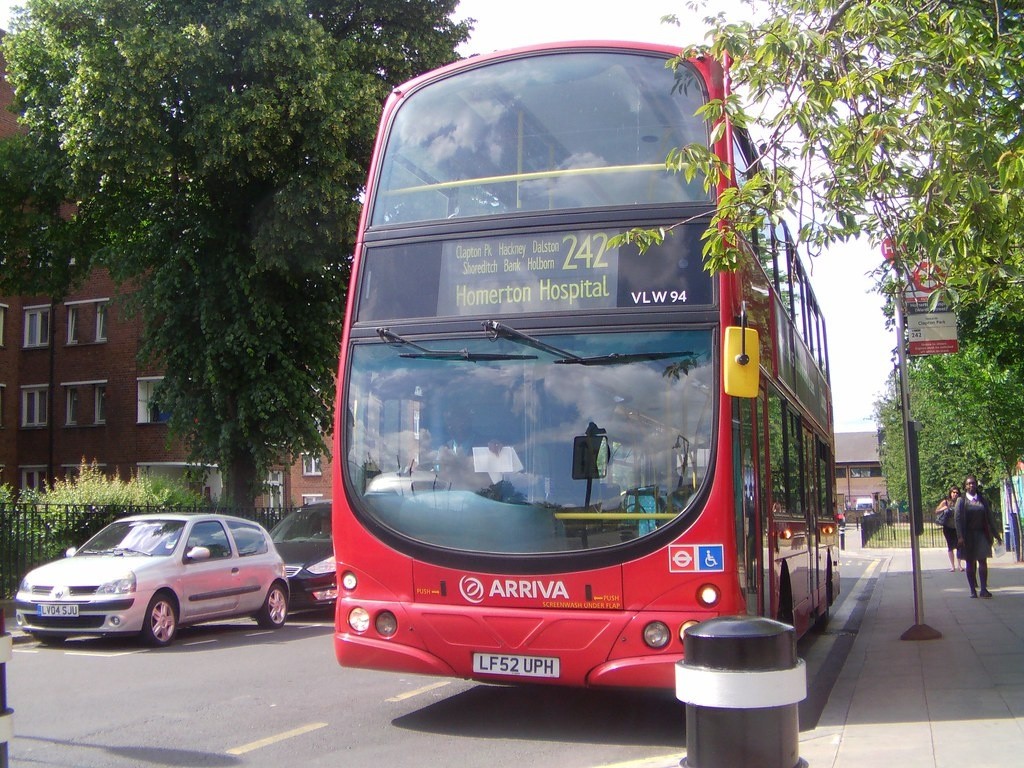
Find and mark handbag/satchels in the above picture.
[935,500,950,527]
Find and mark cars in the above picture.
[268,504,337,608]
[15,513,290,647]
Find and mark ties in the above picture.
[972,498,976,502]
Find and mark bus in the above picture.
[331,41,840,690]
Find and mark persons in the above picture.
[954,476,1001,599]
[936,486,964,572]
[410,408,502,472]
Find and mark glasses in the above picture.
[952,491,959,494]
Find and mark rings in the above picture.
[496,444,498,446]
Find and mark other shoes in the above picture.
[950,567,955,572]
[958,567,964,572]
[980,589,992,597]
[970,592,977,597]
